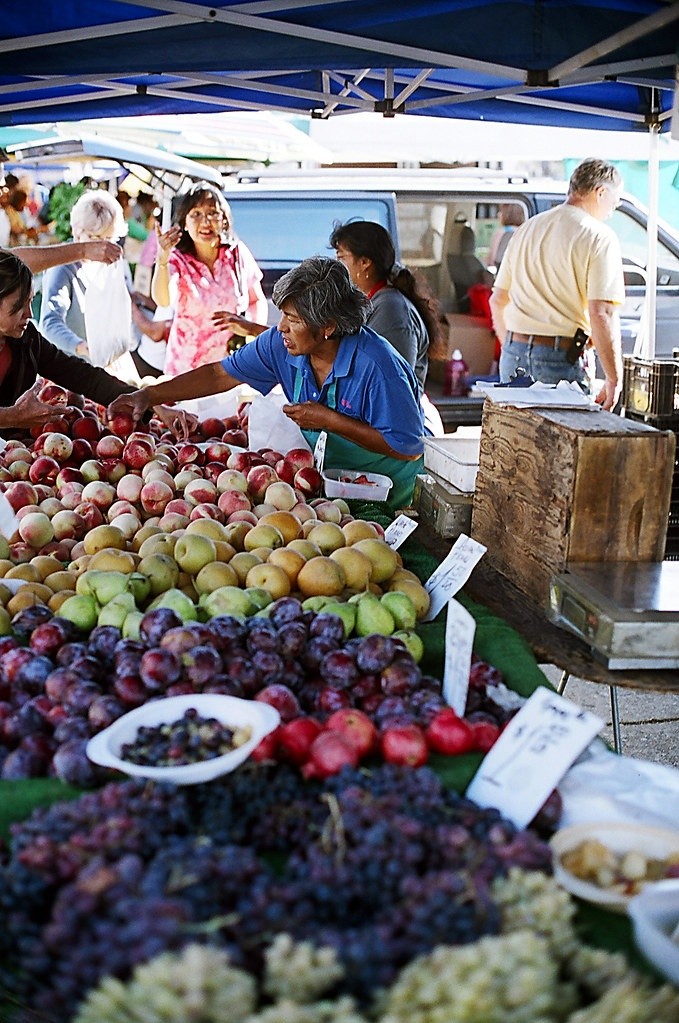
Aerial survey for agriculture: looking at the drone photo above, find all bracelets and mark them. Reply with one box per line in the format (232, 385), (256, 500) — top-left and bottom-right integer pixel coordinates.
(155, 259), (167, 268)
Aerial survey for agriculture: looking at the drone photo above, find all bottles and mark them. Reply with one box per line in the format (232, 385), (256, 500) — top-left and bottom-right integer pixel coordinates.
(443, 349), (468, 397)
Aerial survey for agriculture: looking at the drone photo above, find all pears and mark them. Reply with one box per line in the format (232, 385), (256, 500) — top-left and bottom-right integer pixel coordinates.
(0, 511), (423, 666)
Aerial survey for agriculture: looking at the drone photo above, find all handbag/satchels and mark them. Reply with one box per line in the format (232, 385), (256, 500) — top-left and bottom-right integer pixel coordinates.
(76, 252), (140, 368)
(247, 393), (312, 458)
(225, 245), (246, 356)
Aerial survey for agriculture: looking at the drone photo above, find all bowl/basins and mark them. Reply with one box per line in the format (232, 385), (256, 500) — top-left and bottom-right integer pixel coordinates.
(626, 878), (678, 984)
(548, 822), (679, 914)
(322, 468), (394, 500)
(84, 693), (281, 786)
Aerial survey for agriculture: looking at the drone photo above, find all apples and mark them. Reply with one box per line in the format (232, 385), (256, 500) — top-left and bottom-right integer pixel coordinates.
(253, 684), (501, 772)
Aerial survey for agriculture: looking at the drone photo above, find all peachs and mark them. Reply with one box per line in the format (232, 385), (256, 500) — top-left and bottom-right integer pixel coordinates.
(0, 385), (386, 558)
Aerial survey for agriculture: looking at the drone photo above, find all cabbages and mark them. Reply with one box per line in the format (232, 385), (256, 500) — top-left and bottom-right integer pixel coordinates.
(69, 190), (131, 244)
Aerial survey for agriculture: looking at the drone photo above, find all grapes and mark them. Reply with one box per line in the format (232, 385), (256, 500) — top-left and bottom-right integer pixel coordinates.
(0, 759), (679, 1023)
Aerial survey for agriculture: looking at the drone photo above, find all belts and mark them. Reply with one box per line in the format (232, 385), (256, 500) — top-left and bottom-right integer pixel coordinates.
(506, 331), (574, 349)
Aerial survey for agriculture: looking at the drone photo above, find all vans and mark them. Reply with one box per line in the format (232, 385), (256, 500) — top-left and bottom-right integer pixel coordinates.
(5, 135), (679, 427)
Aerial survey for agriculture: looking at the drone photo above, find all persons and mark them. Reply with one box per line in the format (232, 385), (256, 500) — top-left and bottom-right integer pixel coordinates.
(151, 183), (269, 376)
(0, 175), (36, 248)
(115, 191), (155, 240)
(329, 221), (448, 391)
(0, 247), (198, 441)
(489, 204), (524, 265)
(492, 159), (625, 410)
(41, 189), (166, 367)
(8, 241), (123, 274)
(106, 258), (433, 511)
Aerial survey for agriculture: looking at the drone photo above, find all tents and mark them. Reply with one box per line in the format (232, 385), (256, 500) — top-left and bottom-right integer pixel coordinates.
(1, 0), (679, 367)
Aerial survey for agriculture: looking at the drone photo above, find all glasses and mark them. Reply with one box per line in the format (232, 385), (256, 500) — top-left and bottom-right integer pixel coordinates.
(593, 182), (623, 211)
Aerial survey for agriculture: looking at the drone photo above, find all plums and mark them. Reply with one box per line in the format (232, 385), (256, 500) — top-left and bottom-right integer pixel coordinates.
(464, 658), (525, 724)
(0, 604), (445, 786)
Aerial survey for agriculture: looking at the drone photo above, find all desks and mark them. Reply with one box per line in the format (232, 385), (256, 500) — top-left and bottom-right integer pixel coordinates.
(404, 504), (679, 755)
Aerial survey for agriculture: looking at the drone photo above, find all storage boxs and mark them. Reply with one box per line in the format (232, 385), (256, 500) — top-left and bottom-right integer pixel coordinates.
(412, 354), (679, 608)
